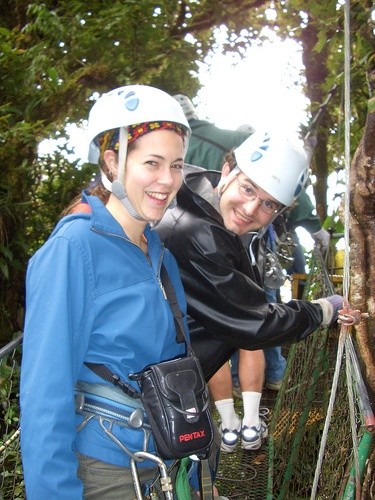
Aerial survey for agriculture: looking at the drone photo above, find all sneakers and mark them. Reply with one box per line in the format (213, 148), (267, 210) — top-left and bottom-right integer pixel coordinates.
(218, 413), (242, 452)
(241, 416), (268, 450)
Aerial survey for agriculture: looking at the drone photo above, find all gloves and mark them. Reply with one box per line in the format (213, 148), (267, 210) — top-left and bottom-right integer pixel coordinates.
(313, 294), (349, 329)
(311, 226), (331, 256)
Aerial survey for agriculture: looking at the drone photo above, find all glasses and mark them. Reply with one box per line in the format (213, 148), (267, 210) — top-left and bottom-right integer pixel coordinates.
(235, 177), (282, 217)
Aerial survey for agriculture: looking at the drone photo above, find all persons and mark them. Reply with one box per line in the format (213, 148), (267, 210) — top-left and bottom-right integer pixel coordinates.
(19, 85), (219, 500)
(150, 128), (348, 386)
(171, 93), (330, 453)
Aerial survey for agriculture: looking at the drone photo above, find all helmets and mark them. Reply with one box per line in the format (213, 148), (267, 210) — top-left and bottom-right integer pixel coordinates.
(235, 124), (309, 209)
(85, 85), (192, 166)
(171, 93), (199, 122)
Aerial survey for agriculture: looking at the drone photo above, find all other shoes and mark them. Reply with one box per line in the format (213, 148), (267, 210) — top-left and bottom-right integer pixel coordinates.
(266, 374), (292, 390)
(232, 386), (243, 399)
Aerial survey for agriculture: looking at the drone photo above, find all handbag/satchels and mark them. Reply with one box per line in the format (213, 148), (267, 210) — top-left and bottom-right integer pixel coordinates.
(129, 352), (214, 461)
